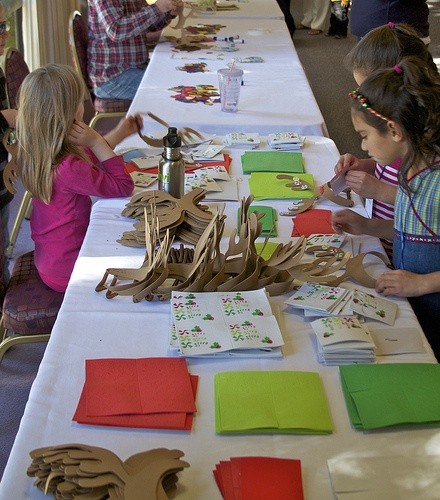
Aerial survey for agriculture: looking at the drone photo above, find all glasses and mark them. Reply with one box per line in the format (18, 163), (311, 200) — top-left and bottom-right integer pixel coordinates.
(0, 23), (11, 34)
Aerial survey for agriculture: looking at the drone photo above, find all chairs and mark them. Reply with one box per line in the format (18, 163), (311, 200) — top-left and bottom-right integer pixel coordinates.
(2, 47), (32, 256)
(68, 11), (127, 129)
(0, 249), (65, 363)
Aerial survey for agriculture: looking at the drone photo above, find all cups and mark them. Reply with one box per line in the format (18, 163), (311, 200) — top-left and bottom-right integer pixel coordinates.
(217, 68), (244, 113)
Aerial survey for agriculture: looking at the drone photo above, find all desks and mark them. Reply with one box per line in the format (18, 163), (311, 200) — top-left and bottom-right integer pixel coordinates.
(0, 137), (440, 500)
(127, 19), (331, 137)
(176, 0), (285, 19)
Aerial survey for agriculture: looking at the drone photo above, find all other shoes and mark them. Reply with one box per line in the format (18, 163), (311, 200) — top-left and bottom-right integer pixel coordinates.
(325, 33), (335, 36)
(295, 24), (311, 30)
(306, 30), (322, 35)
(334, 34), (347, 39)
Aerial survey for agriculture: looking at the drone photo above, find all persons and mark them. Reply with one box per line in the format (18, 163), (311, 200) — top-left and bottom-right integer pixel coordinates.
(335, 21), (435, 265)
(85, 0), (185, 101)
(0, 3), (17, 283)
(327, 57), (440, 296)
(17, 64), (143, 291)
(281, 0), (430, 51)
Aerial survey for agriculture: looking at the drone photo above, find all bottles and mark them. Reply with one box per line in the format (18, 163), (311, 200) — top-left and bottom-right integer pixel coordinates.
(158, 127), (185, 199)
